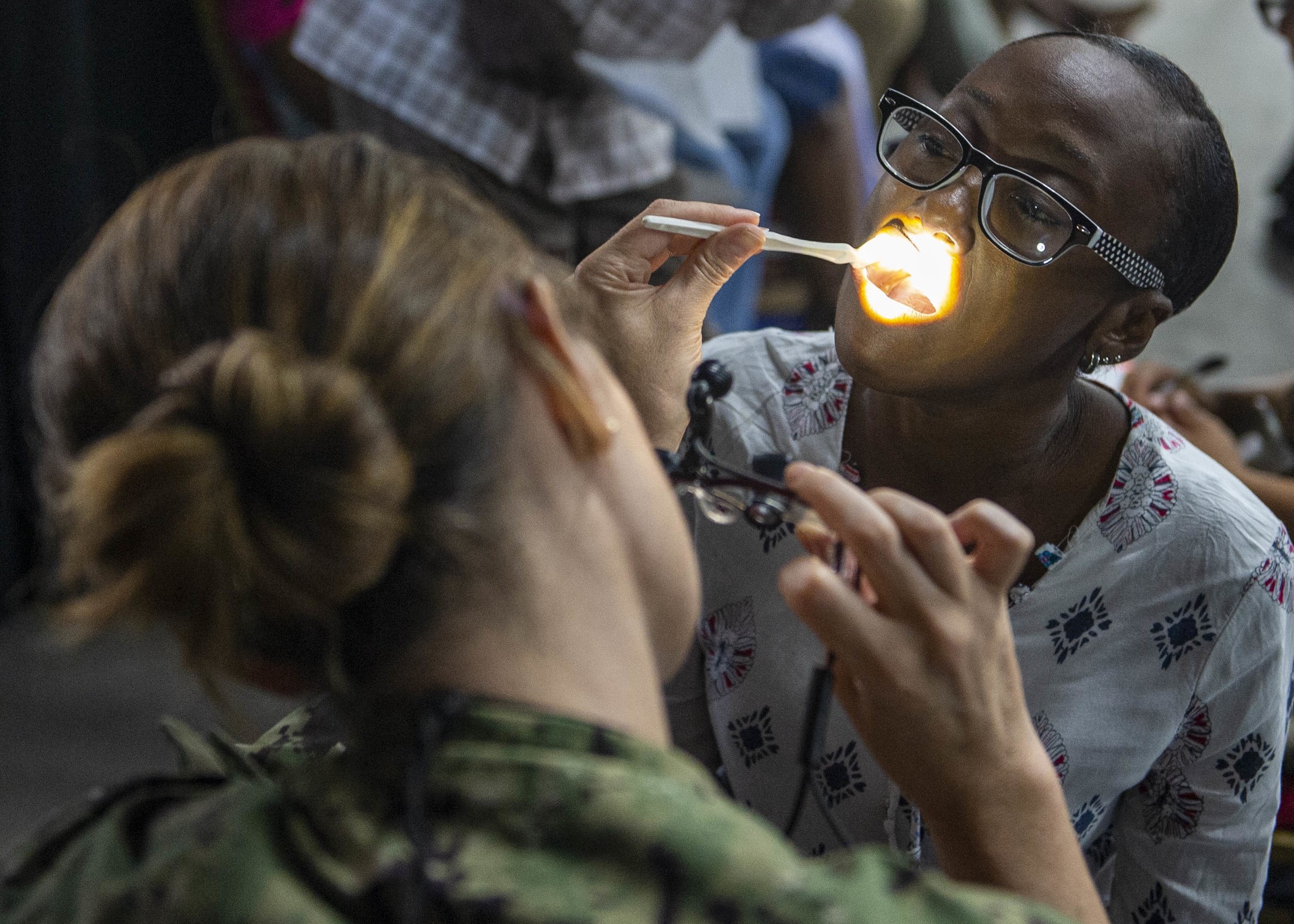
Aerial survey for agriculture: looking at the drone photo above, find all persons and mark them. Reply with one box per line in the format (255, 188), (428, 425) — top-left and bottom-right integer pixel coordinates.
(1, 137), (1113, 924)
(656, 31), (1294, 924)
(290, 3), (1294, 518)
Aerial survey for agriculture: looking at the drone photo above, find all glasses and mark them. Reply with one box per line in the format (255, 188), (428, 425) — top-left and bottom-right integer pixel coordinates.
(875, 86), (1168, 295)
